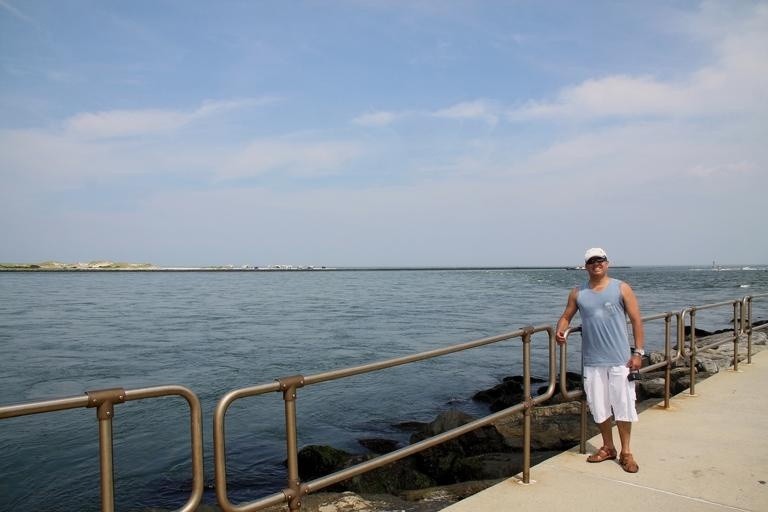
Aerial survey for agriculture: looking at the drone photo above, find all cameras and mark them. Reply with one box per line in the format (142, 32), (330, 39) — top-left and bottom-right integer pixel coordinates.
(627, 373), (642, 382)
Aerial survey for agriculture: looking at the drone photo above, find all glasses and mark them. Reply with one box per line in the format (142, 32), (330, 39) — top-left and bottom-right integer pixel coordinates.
(587, 258), (606, 264)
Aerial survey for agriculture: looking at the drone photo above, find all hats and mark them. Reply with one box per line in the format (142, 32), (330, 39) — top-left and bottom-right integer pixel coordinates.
(584, 247), (608, 264)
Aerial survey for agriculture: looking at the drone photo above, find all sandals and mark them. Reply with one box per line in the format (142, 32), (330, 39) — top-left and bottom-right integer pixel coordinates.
(618, 450), (639, 473)
(586, 446), (617, 463)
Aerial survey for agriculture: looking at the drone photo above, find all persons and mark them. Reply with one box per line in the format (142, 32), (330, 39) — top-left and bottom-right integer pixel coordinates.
(554, 246), (644, 473)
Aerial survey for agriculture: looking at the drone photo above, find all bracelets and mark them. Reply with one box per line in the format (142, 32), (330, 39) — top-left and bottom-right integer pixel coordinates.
(632, 349), (643, 358)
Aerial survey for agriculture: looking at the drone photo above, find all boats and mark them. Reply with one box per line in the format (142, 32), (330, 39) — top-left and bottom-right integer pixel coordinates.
(566, 265), (588, 270)
